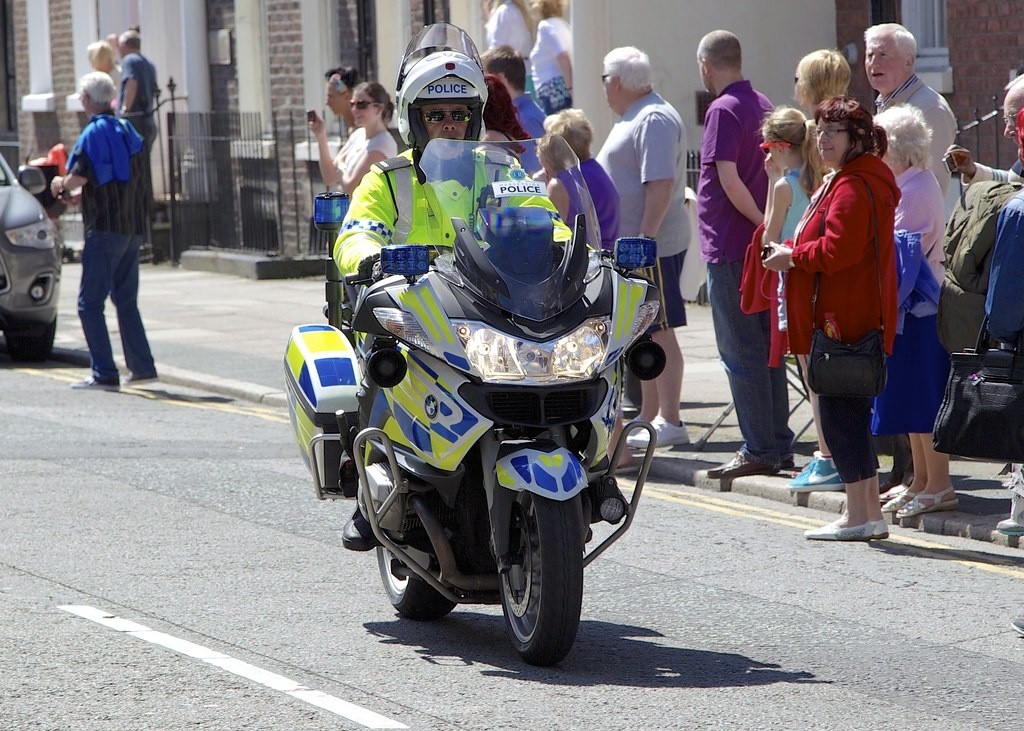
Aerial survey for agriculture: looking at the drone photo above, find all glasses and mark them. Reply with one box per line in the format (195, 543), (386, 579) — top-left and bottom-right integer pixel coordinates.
(601, 74), (609, 83)
(815, 126), (861, 137)
(759, 141), (790, 155)
(349, 100), (380, 109)
(1001, 115), (1018, 127)
(422, 107), (472, 124)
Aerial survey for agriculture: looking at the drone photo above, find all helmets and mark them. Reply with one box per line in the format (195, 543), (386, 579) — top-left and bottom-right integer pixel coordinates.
(396, 22), (488, 149)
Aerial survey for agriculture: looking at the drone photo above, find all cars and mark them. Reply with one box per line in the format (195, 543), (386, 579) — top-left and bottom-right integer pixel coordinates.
(1, 154), (61, 366)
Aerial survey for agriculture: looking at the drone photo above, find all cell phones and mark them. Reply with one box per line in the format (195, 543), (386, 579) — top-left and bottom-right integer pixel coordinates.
(761, 245), (775, 260)
(942, 152), (964, 174)
(308, 111), (316, 122)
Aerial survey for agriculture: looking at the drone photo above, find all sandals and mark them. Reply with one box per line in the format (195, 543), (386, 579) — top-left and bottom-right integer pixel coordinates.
(882, 489), (921, 512)
(895, 485), (958, 518)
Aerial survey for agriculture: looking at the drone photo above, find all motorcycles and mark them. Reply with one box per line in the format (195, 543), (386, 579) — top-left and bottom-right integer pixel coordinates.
(284, 132), (667, 664)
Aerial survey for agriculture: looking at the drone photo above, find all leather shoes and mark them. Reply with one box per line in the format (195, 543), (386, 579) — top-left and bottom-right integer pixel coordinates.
(706, 449), (795, 480)
(342, 508), (376, 551)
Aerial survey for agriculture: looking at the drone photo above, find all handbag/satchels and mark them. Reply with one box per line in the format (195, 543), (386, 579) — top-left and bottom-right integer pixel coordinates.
(805, 327), (885, 399)
(932, 315), (1024, 462)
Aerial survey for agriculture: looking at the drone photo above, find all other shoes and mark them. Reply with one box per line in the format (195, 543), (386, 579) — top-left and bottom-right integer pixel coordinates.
(804, 516), (873, 540)
(71, 376), (121, 392)
(869, 519), (889, 538)
(119, 368), (160, 385)
(880, 480), (912, 502)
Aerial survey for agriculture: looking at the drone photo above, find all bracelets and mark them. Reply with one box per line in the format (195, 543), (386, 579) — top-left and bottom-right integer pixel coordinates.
(61, 177), (70, 193)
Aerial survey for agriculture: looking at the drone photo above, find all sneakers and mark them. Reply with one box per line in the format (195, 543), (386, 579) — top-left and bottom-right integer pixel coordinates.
(786, 450), (845, 491)
(997, 520), (1024, 535)
(626, 415), (687, 447)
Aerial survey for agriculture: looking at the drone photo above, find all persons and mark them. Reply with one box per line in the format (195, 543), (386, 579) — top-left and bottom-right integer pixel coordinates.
(943, 69), (1024, 537)
(86, 30), (158, 235)
(694, 30), (796, 479)
(595, 47), (690, 448)
(761, 24), (958, 540)
(332, 23), (629, 552)
(51, 72), (159, 389)
(306, 0), (622, 471)
